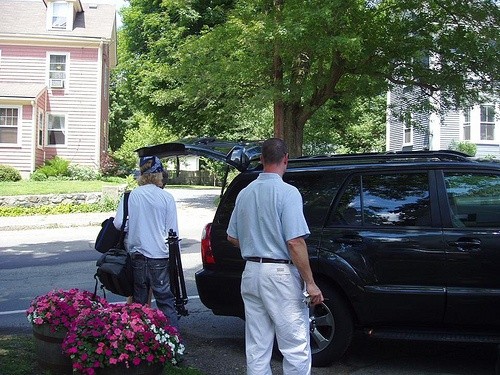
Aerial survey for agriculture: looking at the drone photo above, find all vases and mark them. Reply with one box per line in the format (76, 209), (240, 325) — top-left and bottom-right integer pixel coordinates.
(96, 358), (164, 375)
(32, 322), (73, 371)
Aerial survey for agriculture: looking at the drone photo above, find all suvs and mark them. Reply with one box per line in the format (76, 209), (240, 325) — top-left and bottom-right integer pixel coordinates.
(134, 136), (500, 367)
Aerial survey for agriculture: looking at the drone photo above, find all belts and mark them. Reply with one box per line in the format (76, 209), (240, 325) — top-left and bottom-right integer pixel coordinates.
(247, 256), (294, 264)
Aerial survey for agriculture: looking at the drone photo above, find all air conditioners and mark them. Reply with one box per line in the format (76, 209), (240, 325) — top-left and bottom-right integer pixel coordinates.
(50, 79), (63, 88)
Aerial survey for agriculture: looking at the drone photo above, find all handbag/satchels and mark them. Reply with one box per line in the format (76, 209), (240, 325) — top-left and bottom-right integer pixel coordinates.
(94, 217), (126, 254)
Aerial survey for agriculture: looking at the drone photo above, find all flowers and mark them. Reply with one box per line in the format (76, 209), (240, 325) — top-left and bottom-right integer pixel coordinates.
(26, 287), (108, 328)
(61, 301), (185, 375)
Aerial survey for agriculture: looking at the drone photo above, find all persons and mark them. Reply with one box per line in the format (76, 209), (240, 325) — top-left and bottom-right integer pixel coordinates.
(114, 155), (181, 330)
(226, 139), (324, 375)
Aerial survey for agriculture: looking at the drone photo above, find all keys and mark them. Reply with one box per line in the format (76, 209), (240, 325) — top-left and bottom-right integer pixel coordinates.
(302, 292), (312, 307)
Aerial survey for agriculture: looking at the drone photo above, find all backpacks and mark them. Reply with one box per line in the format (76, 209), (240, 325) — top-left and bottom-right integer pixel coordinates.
(97, 248), (133, 297)
